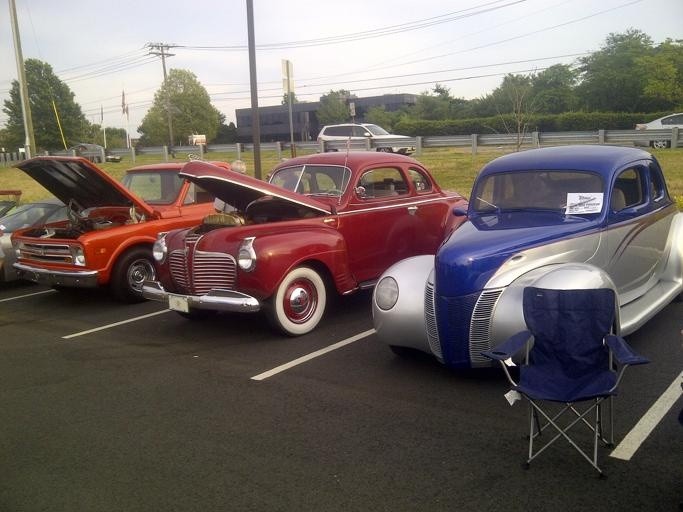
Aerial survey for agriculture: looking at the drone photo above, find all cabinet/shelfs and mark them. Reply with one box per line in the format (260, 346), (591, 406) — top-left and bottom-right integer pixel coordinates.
(366, 183), (398, 196)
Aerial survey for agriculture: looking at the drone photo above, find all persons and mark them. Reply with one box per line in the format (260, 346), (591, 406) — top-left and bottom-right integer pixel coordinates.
(212, 159), (247, 214)
(0, 224), (6, 270)
(277, 157), (304, 195)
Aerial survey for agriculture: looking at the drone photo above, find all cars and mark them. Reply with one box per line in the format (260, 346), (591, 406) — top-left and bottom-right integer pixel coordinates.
(632, 112), (683, 148)
(373, 142), (683, 366)
(8, 156), (232, 302)
(0, 198), (65, 285)
(141, 151), (468, 337)
(0, 189), (22, 220)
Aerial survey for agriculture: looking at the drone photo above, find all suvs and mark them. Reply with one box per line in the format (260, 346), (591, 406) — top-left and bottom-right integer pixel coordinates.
(316, 122), (415, 155)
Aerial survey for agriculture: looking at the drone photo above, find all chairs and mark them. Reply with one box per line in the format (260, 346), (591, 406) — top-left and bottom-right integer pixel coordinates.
(611, 189), (626, 212)
(480, 287), (651, 479)
(516, 177), (547, 205)
